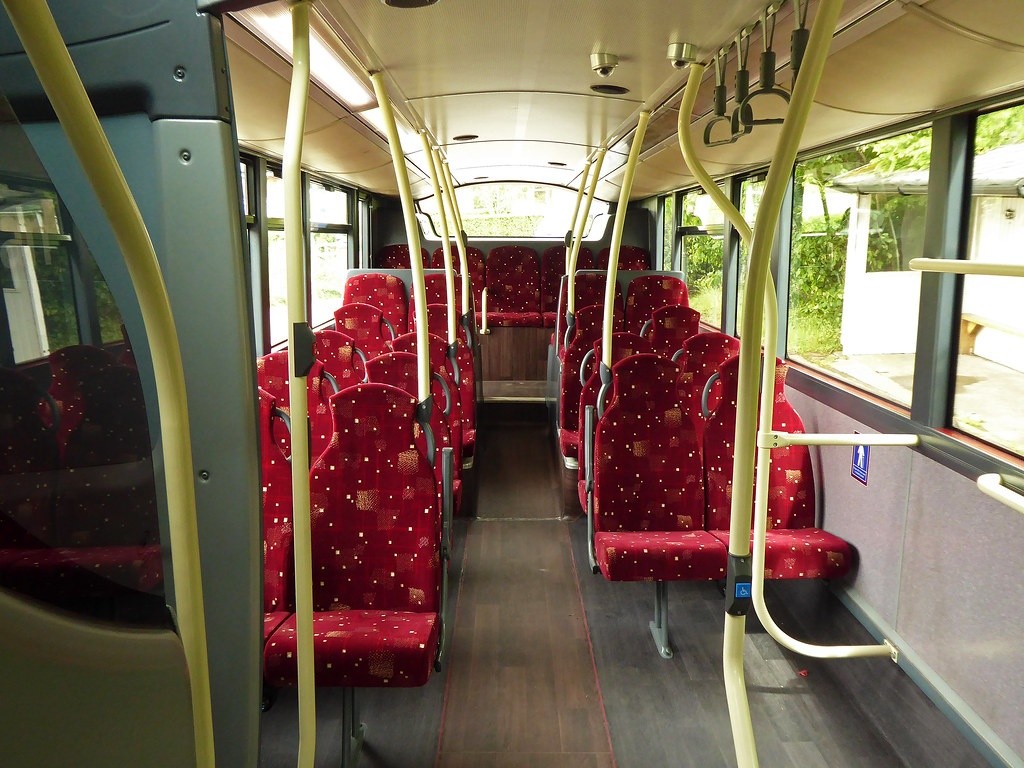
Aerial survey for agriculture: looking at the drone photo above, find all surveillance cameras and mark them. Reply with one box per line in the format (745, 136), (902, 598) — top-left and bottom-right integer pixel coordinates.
(667, 43), (690, 69)
(590, 53), (618, 78)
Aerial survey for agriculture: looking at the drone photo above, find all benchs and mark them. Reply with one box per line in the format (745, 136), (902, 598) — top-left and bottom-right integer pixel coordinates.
(258, 244), (856, 768)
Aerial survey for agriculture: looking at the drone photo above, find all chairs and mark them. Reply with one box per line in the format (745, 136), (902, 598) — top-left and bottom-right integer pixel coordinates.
(0, 322), (166, 628)
